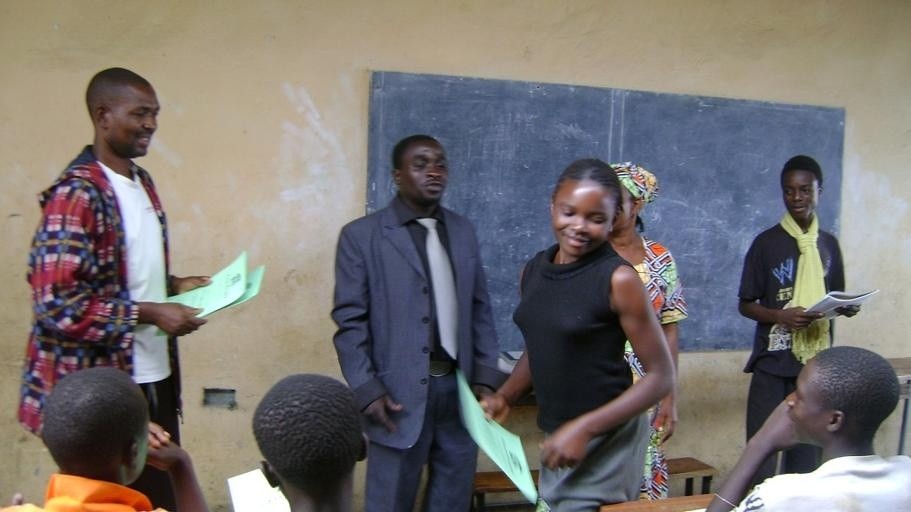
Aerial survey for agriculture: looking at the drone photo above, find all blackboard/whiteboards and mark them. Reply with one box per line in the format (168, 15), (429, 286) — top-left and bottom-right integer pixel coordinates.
(365, 71), (846, 353)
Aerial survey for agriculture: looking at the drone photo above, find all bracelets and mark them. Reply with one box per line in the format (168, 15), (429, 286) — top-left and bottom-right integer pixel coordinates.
(713, 491), (737, 508)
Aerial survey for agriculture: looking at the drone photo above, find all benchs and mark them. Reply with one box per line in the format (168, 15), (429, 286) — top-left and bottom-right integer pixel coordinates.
(469, 456), (715, 510)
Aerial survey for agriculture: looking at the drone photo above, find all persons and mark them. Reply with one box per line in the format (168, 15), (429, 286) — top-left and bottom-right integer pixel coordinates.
(481, 159), (676, 512)
(605, 161), (687, 499)
(738, 156), (882, 500)
(18, 67), (211, 507)
(704, 346), (911, 512)
(329, 134), (499, 512)
(0, 370), (368, 512)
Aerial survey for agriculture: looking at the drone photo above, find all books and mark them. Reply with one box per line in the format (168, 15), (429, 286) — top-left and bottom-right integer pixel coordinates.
(805, 288), (881, 328)
(153, 251), (264, 336)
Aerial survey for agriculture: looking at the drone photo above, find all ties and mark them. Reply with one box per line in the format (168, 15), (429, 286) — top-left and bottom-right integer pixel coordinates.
(414, 217), (462, 360)
